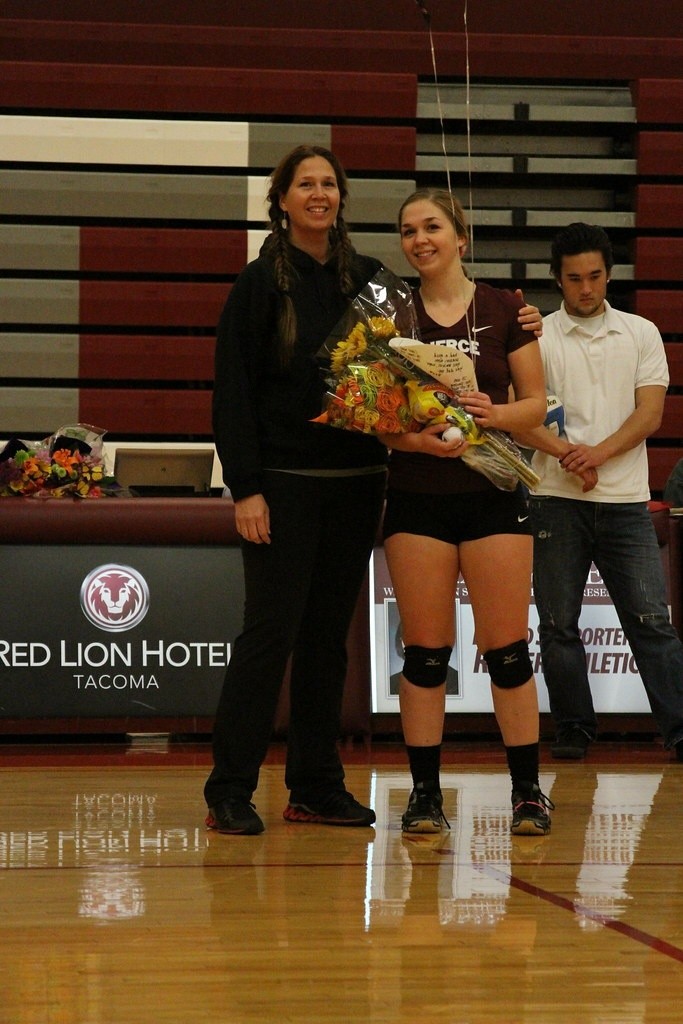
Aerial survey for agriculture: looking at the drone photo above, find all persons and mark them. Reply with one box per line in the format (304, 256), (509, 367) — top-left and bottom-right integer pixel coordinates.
(509, 221), (683, 765)
(203, 145), (542, 835)
(376, 190), (548, 834)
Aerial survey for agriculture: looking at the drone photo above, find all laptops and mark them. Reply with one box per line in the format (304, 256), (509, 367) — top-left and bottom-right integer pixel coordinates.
(114, 448), (215, 498)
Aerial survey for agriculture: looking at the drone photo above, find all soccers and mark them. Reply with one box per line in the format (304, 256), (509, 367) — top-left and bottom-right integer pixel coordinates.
(511, 388), (565, 451)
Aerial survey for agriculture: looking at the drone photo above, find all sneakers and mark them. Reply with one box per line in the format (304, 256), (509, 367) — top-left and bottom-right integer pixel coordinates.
(283, 788), (376, 825)
(400, 779), (451, 832)
(550, 726), (589, 758)
(511, 781), (557, 835)
(205, 796), (265, 833)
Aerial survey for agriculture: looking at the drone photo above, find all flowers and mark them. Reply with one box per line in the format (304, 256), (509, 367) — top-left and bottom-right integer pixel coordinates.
(304, 309), (550, 500)
(0, 429), (113, 500)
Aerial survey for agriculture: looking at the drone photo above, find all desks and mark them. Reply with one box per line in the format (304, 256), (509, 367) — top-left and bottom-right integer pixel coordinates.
(0, 498), (372, 748)
